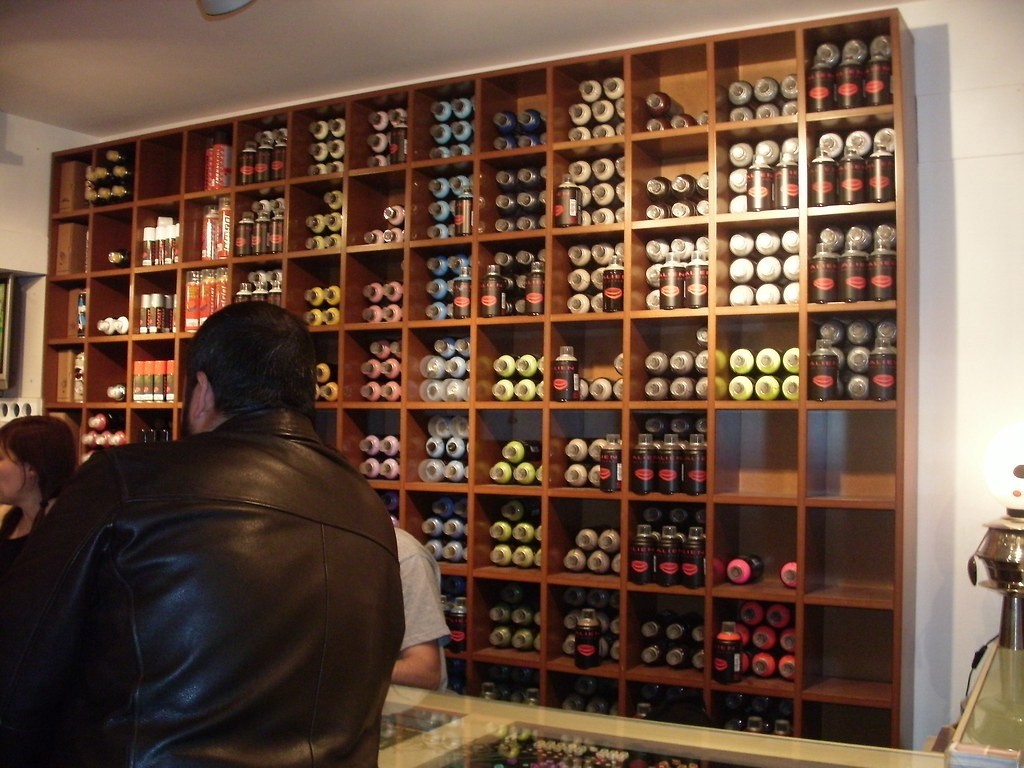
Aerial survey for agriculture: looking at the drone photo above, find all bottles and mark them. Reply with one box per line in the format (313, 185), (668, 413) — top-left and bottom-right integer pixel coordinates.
(713, 692), (793, 738)
(551, 673), (619, 718)
(712, 598), (796, 686)
(69, 36), (899, 501)
(639, 619), (706, 673)
(633, 505), (708, 590)
(562, 527), (623, 574)
(558, 587), (622, 669)
(780, 562), (798, 587)
(434, 576), (467, 653)
(488, 499), (542, 569)
(373, 488), (399, 526)
(418, 495), (468, 565)
(727, 553), (764, 584)
(483, 582), (541, 652)
(473, 664), (542, 708)
(633, 685), (705, 726)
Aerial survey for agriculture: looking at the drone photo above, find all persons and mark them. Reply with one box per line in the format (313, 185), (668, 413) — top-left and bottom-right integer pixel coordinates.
(386, 519), (451, 695)
(0, 299), (408, 768)
(0, 414), (80, 671)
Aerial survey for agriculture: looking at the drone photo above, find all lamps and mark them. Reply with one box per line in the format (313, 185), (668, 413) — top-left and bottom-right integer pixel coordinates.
(967, 462), (1024, 650)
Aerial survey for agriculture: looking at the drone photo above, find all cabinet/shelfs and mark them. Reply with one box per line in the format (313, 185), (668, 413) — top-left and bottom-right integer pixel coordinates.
(39, 6), (918, 749)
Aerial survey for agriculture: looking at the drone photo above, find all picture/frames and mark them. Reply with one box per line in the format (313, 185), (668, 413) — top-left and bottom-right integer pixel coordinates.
(0, 272), (15, 390)
